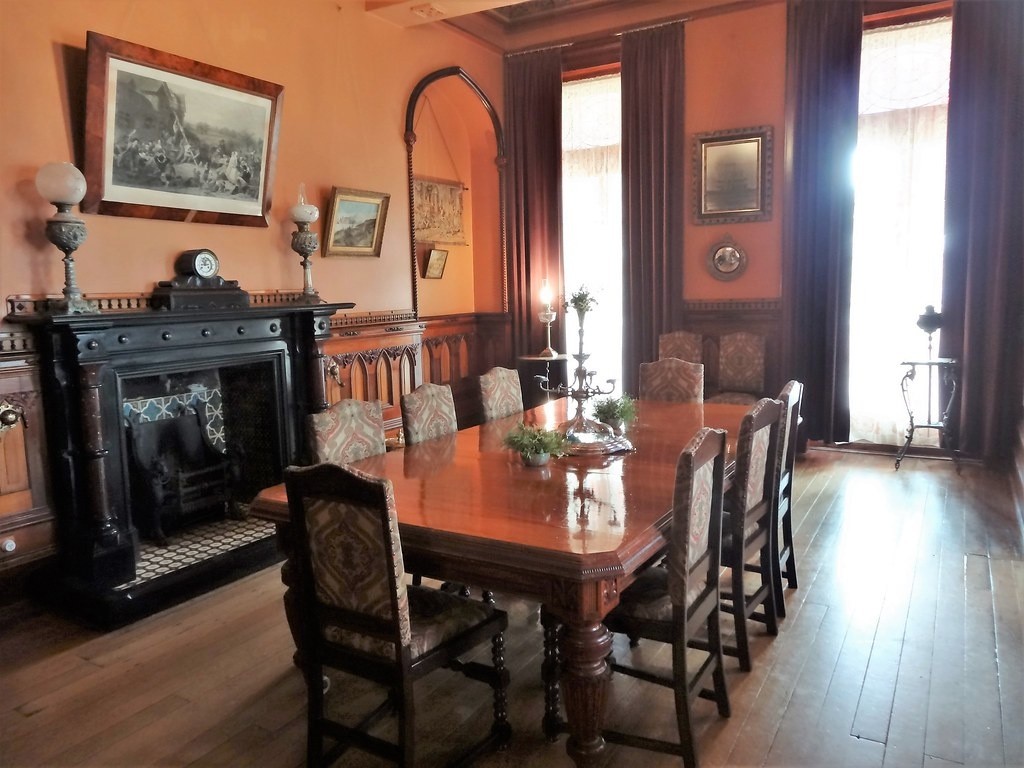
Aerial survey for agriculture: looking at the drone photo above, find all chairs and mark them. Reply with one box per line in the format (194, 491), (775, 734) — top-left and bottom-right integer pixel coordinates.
(604, 426), (730, 767)
(719, 398), (784, 671)
(639, 358), (703, 403)
(658, 329), (703, 364)
(773, 380), (806, 620)
(704, 331), (765, 405)
(281, 462), (507, 768)
(476, 367), (523, 421)
(400, 383), (457, 446)
(310, 397), (386, 466)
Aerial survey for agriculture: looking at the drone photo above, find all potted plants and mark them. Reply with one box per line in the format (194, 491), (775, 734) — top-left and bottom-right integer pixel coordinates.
(501, 422), (568, 466)
(561, 283), (598, 352)
(595, 392), (641, 426)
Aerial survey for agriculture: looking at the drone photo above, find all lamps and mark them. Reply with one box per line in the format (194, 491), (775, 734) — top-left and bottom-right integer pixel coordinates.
(535, 288), (559, 358)
(289, 180), (328, 305)
(35, 158), (101, 314)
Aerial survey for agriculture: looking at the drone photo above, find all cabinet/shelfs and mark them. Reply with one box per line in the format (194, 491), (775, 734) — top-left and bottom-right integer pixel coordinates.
(889, 359), (966, 475)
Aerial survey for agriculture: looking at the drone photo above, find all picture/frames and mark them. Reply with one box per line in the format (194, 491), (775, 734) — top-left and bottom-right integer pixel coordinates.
(690, 123), (773, 226)
(321, 185), (391, 258)
(422, 249), (449, 279)
(80, 31), (283, 228)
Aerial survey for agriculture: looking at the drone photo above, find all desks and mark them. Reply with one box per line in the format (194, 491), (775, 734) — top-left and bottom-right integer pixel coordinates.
(249, 396), (756, 768)
(518, 354), (571, 401)
